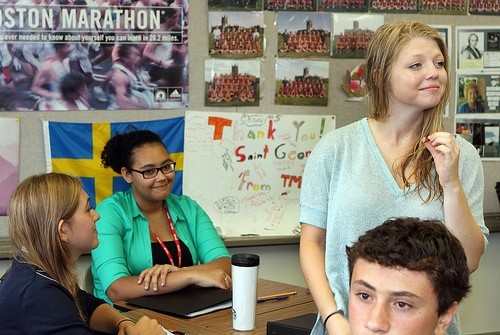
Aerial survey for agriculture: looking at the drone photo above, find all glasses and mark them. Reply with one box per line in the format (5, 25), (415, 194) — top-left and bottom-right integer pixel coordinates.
(126, 160), (176, 179)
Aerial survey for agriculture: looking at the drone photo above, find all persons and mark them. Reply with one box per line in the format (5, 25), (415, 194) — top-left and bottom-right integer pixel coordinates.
(0, 172), (167, 335)
(0, 0), (486, 114)
(298, 21), (492, 335)
(90, 130), (232, 307)
(345, 216), (472, 335)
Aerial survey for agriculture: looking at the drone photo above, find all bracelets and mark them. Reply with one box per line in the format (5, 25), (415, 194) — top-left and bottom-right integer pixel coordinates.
(323, 310), (344, 332)
(117, 319), (136, 331)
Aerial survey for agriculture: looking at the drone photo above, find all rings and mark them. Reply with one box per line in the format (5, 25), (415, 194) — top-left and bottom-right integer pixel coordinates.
(224, 278), (228, 280)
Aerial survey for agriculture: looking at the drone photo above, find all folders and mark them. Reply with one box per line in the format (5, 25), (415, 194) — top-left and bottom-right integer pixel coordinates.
(125, 283), (234, 320)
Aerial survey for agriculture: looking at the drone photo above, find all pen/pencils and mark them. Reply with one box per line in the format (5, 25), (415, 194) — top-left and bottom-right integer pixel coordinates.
(242, 234), (259, 237)
(256, 290), (297, 303)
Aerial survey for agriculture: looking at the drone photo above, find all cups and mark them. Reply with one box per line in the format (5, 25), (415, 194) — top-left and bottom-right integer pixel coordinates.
(231, 253), (260, 331)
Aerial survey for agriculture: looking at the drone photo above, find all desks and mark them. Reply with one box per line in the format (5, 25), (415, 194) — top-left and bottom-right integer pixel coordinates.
(112, 278), (318, 335)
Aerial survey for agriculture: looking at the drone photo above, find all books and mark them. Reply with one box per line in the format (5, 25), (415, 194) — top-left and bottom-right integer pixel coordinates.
(126, 284), (232, 318)
